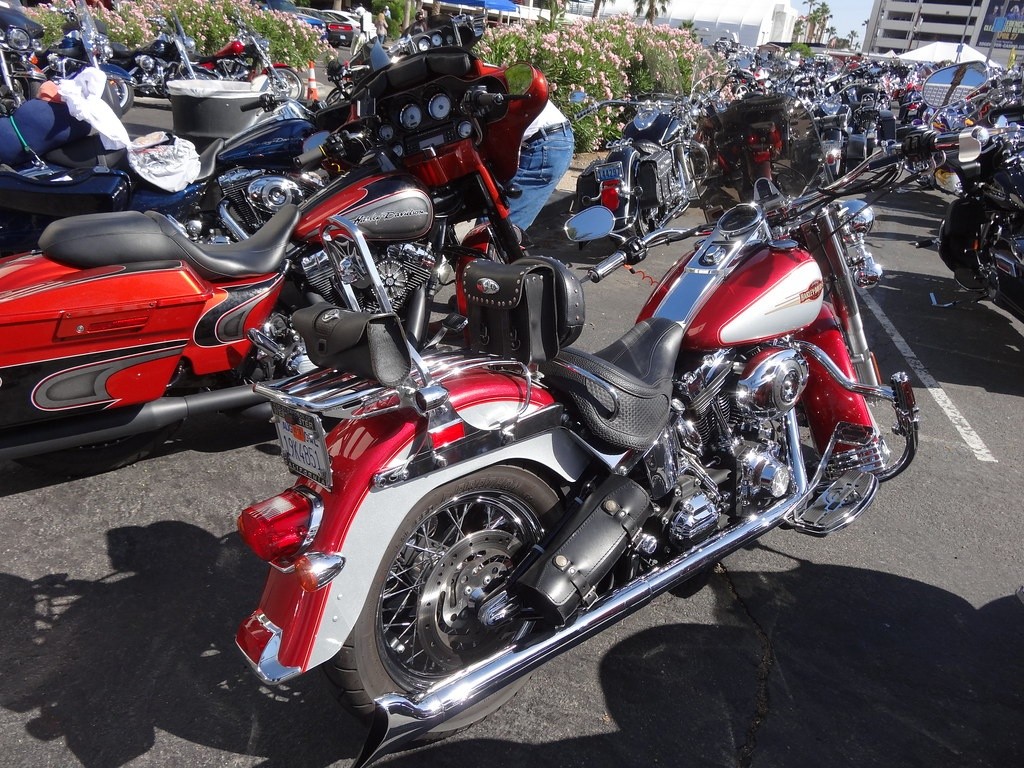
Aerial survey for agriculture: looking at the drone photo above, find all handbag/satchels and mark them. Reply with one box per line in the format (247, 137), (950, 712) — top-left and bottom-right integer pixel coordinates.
(291, 301), (410, 385)
(127, 130), (201, 193)
(462, 258), (560, 364)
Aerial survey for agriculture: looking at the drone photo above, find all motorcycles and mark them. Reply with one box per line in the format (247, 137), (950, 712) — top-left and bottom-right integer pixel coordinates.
(230, 149), (922, 768)
(0, 1), (1024, 470)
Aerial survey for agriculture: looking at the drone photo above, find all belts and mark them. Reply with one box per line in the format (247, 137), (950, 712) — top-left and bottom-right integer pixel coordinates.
(525, 123), (570, 144)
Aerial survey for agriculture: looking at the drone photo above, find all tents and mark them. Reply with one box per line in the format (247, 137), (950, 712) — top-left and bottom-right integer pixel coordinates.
(863, 50), (899, 59)
(438, 0), (516, 25)
(900, 42), (999, 68)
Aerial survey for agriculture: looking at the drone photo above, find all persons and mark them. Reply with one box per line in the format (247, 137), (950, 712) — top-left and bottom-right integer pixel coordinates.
(475, 99), (575, 232)
(986, 5), (1024, 27)
(377, 12), (388, 44)
(383, 6), (390, 18)
(355, 3), (366, 16)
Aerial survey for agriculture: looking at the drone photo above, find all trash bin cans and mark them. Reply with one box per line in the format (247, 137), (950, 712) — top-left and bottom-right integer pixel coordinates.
(166, 79), (274, 156)
